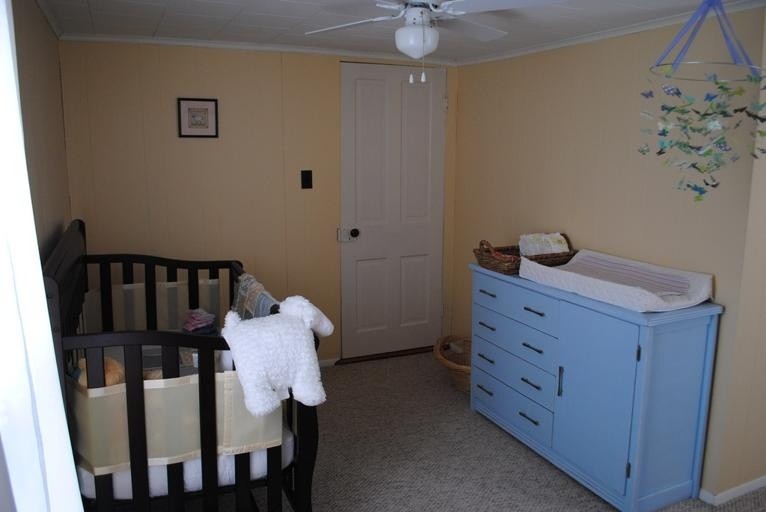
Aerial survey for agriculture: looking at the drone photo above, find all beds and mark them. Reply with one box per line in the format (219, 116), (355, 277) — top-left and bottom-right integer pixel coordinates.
(40, 213), (324, 511)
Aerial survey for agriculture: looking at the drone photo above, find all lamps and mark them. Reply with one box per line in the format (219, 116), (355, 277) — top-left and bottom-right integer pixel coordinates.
(395, 3), (446, 87)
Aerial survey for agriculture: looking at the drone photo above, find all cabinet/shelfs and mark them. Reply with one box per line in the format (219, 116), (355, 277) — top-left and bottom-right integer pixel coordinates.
(467, 262), (564, 465)
(549, 290), (724, 512)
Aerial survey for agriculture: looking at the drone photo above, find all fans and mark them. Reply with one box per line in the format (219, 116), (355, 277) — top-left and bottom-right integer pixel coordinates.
(298, 0), (509, 48)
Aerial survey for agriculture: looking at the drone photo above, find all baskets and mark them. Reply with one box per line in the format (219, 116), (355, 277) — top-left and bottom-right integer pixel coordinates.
(429, 330), (474, 396)
(469, 227), (582, 278)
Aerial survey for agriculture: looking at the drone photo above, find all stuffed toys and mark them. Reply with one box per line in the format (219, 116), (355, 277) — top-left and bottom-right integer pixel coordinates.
(78, 356), (165, 391)
(224, 294), (334, 416)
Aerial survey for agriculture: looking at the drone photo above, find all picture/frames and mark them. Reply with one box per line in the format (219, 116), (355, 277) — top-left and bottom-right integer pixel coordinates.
(175, 97), (220, 139)
